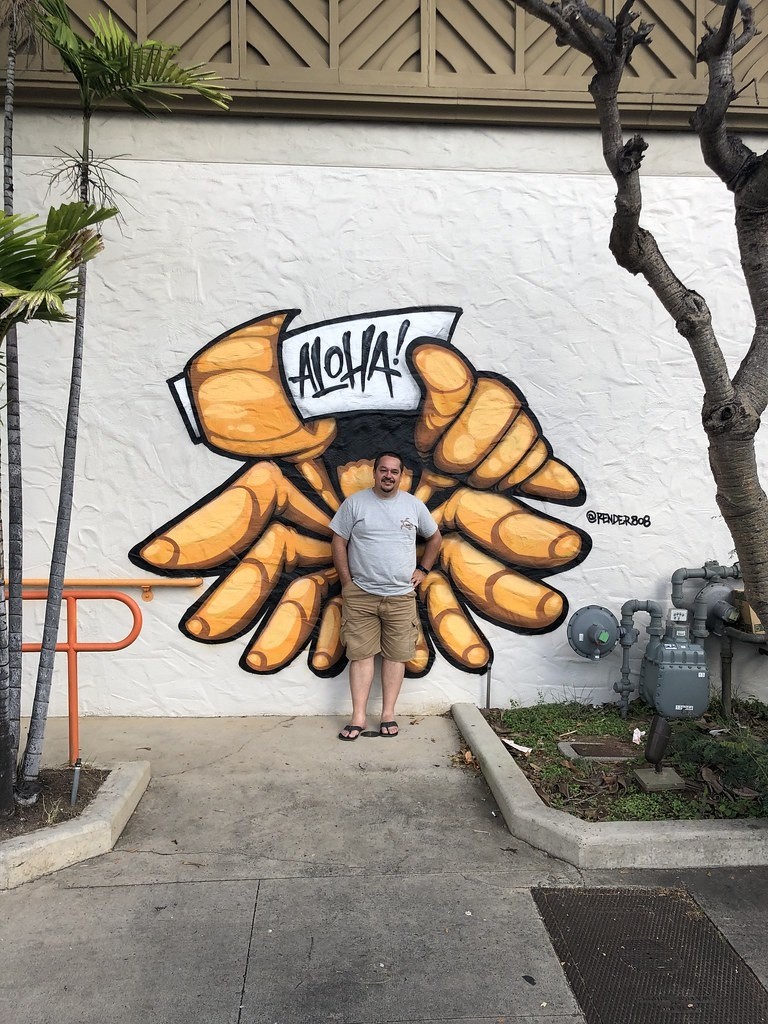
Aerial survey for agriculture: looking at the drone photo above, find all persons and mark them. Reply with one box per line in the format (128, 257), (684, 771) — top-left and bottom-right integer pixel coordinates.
(327, 451), (443, 741)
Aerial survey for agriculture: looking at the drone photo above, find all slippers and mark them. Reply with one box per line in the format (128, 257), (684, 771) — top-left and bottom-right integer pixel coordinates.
(379, 721), (399, 737)
(338, 725), (365, 741)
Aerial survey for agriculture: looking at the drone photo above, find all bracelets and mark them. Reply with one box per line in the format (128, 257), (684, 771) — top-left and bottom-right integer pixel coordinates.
(417, 564), (429, 575)
